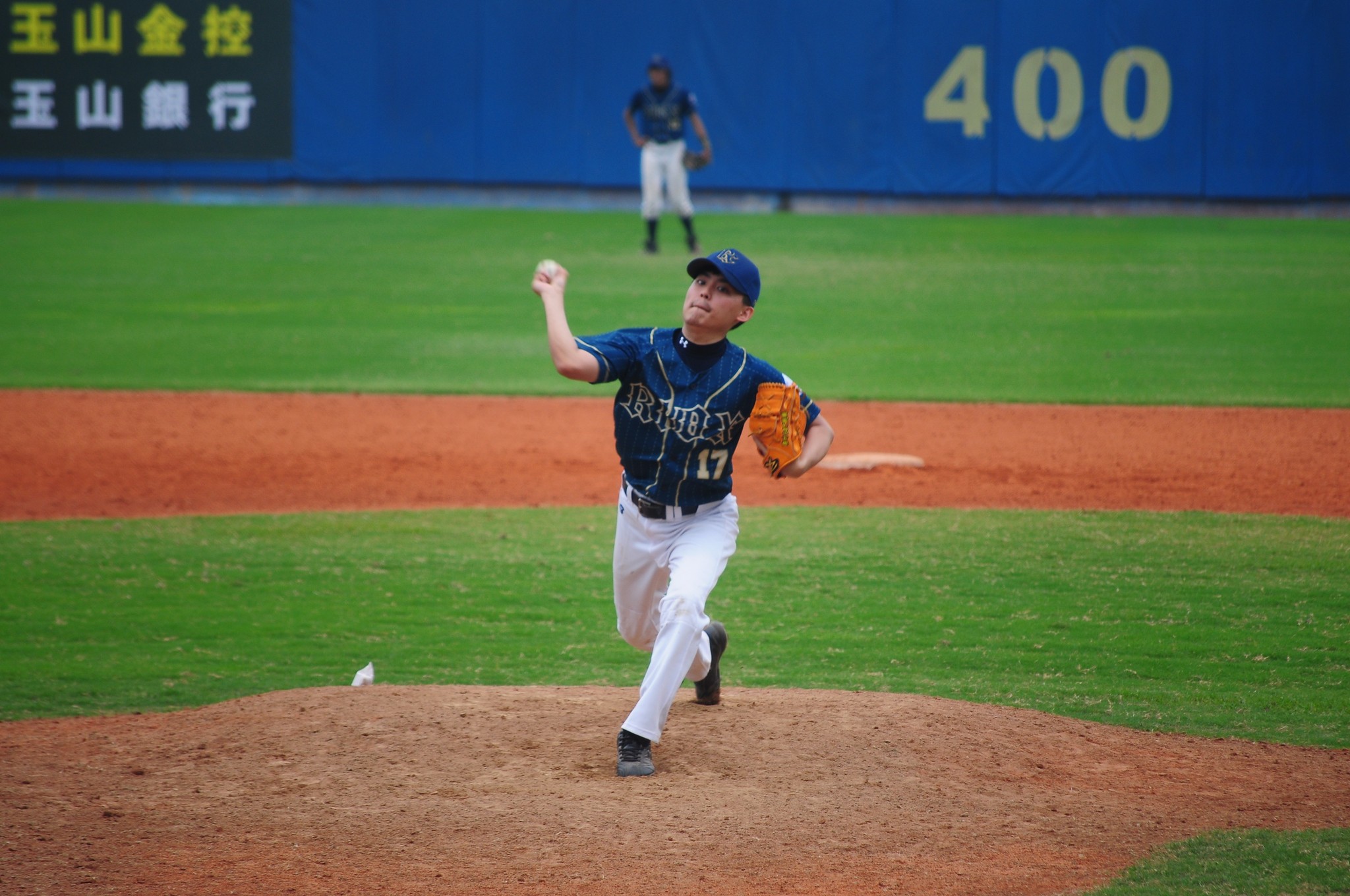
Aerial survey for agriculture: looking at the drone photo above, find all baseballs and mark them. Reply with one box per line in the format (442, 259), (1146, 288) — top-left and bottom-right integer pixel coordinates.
(535, 258), (560, 278)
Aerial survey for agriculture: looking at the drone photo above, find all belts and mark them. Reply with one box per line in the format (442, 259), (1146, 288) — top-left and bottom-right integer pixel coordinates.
(622, 473), (698, 519)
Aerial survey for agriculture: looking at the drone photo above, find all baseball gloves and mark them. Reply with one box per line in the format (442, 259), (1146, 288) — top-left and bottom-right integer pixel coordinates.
(748, 382), (807, 480)
(680, 151), (708, 170)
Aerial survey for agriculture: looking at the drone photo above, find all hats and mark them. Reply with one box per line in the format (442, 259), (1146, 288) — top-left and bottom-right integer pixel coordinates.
(686, 248), (761, 311)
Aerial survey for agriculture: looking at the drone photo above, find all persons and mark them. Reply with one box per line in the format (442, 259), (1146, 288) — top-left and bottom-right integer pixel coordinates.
(529, 249), (834, 778)
(623, 55), (712, 256)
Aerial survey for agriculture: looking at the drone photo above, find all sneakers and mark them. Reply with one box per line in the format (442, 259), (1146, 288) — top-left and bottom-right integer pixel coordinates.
(693, 622), (727, 705)
(615, 729), (656, 776)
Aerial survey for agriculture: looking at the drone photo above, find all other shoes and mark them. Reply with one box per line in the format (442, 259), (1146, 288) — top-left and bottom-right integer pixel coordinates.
(686, 236), (697, 252)
(644, 236), (656, 253)
